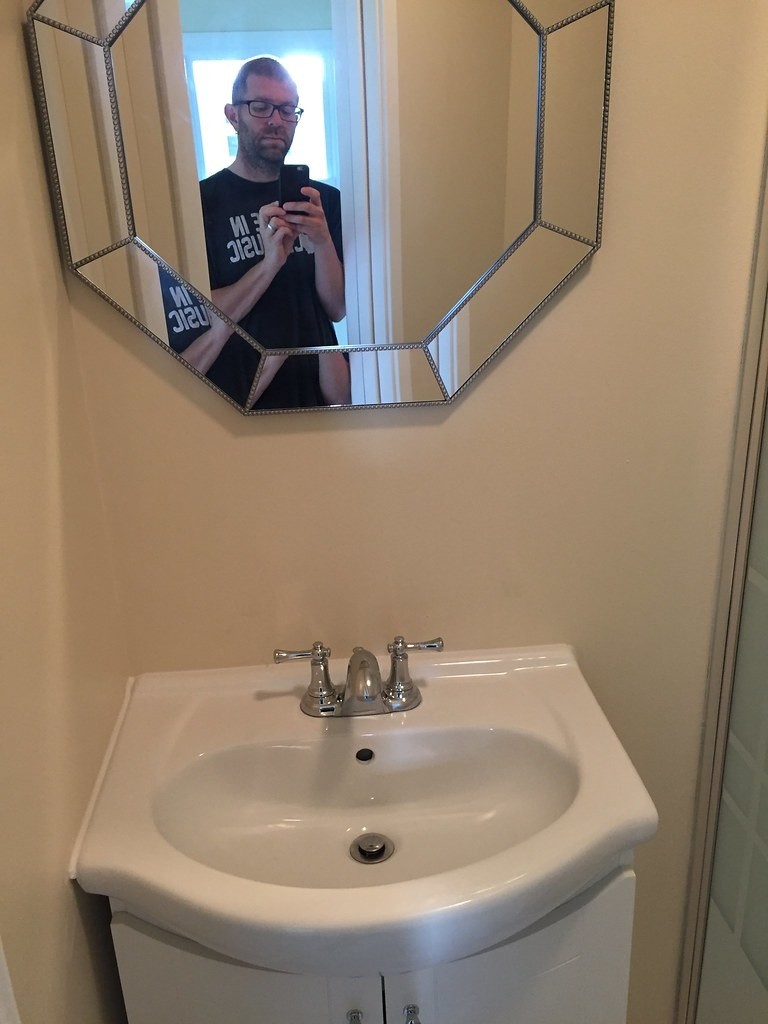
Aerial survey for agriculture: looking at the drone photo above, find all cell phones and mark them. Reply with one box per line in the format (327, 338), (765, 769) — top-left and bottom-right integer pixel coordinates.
(279, 165), (310, 218)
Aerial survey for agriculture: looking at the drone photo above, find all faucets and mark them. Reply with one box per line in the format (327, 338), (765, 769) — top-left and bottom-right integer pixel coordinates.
(344, 645), (385, 718)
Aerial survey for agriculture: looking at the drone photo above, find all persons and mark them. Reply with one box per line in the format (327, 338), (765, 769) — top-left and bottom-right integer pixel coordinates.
(178, 312), (354, 409)
(181, 56), (346, 347)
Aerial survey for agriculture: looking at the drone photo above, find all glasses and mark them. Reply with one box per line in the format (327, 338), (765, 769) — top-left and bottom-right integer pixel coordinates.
(233, 100), (304, 123)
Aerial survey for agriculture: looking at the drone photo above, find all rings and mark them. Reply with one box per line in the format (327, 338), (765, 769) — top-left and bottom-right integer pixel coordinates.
(266, 222), (276, 234)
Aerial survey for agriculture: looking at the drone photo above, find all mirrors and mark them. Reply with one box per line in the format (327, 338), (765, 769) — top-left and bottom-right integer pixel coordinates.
(24, 0), (615, 417)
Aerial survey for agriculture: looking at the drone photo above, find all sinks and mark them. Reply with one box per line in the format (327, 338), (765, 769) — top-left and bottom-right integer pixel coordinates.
(148, 724), (581, 889)
